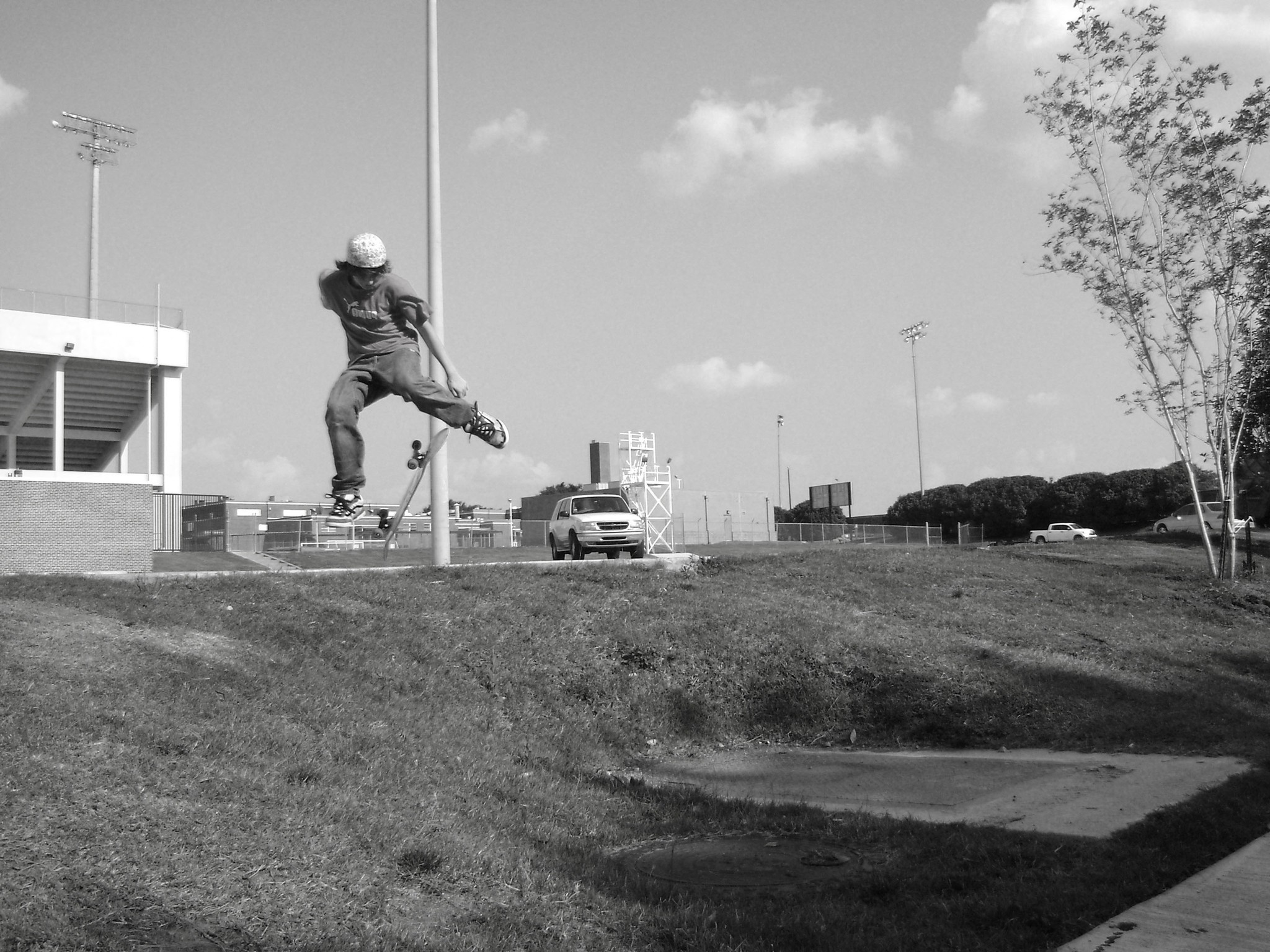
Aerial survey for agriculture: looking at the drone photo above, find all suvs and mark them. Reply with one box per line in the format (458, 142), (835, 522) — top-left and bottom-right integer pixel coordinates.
(549, 494), (645, 560)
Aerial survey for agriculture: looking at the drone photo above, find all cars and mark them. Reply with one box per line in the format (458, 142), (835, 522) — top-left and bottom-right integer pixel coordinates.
(1153, 500), (1230, 534)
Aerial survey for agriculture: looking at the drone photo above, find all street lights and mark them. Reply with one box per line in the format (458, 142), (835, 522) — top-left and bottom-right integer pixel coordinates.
(766, 497), (771, 541)
(53, 111), (138, 320)
(704, 495), (710, 544)
(777, 414), (784, 508)
(897, 316), (930, 498)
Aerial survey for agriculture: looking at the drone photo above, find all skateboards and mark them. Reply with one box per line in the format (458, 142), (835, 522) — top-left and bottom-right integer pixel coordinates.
(375, 428), (451, 560)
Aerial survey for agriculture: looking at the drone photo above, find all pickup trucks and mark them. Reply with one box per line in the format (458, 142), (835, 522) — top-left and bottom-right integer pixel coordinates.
(1029, 522), (1098, 544)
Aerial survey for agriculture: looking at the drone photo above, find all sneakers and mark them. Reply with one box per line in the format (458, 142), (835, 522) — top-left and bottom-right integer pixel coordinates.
(325, 494), (366, 527)
(462, 401), (509, 449)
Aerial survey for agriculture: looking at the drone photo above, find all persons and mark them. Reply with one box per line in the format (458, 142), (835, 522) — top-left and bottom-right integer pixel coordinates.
(319, 231), (510, 528)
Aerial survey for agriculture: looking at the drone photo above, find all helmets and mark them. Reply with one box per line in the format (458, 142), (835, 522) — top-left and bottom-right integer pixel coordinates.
(347, 233), (387, 268)
